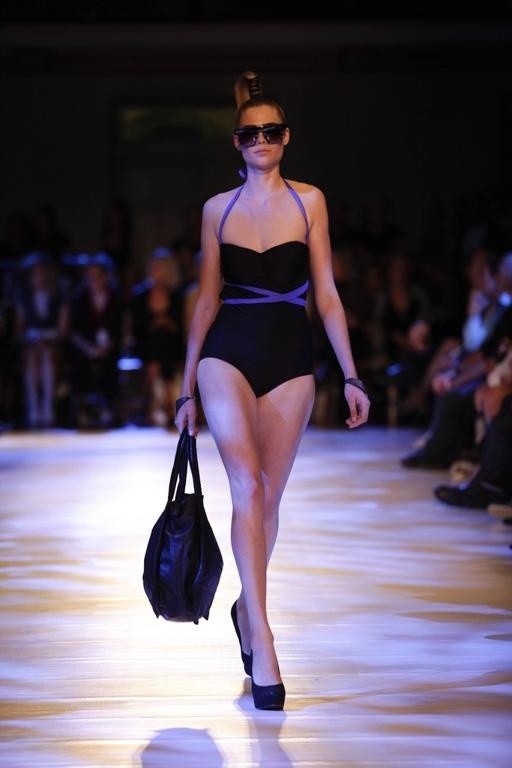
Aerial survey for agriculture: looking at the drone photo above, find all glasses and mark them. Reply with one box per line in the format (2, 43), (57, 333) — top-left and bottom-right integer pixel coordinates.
(234, 124), (287, 147)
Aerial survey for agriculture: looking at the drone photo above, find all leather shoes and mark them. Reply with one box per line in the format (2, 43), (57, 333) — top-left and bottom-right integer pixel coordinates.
(403, 447), (452, 469)
(435, 482), (489, 506)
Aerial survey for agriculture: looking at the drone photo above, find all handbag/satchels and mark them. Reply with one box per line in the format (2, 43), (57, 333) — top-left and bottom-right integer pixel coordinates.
(142, 427), (224, 625)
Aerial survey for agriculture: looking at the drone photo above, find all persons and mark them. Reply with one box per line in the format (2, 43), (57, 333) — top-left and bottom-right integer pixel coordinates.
(4, 242), (434, 430)
(172, 68), (375, 713)
(401, 243), (512, 547)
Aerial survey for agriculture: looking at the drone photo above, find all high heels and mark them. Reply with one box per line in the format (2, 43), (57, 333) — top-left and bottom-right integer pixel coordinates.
(231, 599), (286, 709)
